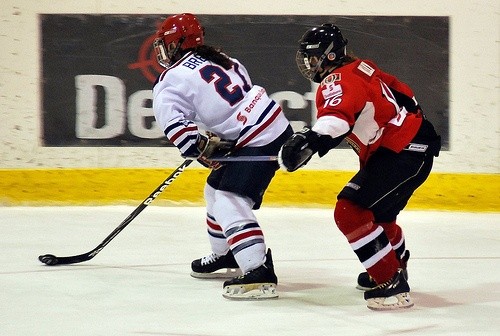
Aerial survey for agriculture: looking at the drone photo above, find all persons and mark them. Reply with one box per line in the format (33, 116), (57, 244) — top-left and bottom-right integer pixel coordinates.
(277, 24), (442, 311)
(152, 14), (294, 299)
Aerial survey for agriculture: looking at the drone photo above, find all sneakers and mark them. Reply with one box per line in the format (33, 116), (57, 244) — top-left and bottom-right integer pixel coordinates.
(362, 268), (413, 311)
(355, 250), (411, 291)
(219, 247), (278, 301)
(191, 249), (243, 280)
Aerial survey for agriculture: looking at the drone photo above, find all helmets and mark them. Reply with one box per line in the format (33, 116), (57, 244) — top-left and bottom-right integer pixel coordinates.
(152, 13), (205, 68)
(295, 21), (350, 84)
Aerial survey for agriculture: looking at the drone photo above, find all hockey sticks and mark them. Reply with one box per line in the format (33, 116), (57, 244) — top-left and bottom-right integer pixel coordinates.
(38, 158), (194, 267)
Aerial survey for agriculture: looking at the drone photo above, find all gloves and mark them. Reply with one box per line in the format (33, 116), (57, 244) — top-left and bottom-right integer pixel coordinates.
(278, 127), (321, 171)
(206, 141), (236, 165)
